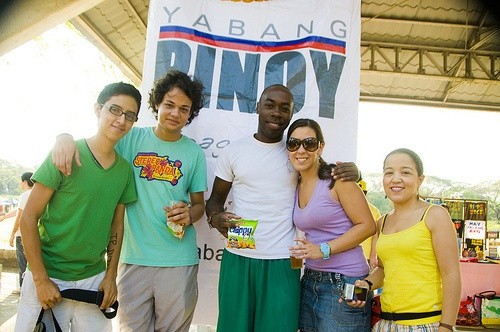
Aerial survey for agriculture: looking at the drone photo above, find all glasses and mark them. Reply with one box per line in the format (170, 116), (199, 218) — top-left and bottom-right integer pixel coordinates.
(285, 137), (321, 153)
(101, 102), (139, 123)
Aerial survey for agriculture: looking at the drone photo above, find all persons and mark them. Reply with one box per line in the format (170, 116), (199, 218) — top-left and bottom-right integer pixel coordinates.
(337, 148), (462, 332)
(12, 82), (135, 332)
(0, 171), (39, 294)
(205, 85), (371, 332)
(51, 68), (209, 332)
(286, 116), (375, 332)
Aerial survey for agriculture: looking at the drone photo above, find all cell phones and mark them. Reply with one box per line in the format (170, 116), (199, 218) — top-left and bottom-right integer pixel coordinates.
(344, 283), (368, 302)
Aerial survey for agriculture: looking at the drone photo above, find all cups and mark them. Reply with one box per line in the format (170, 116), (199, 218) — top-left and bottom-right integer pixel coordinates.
(289, 247), (303, 269)
(167, 200), (184, 223)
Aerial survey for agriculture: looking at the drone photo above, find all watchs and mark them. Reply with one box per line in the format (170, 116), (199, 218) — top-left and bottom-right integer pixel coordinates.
(319, 242), (331, 261)
(439, 322), (456, 332)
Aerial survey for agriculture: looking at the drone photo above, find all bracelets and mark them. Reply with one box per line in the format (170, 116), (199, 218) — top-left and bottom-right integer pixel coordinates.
(207, 210), (221, 229)
(357, 171), (363, 182)
(362, 278), (373, 290)
